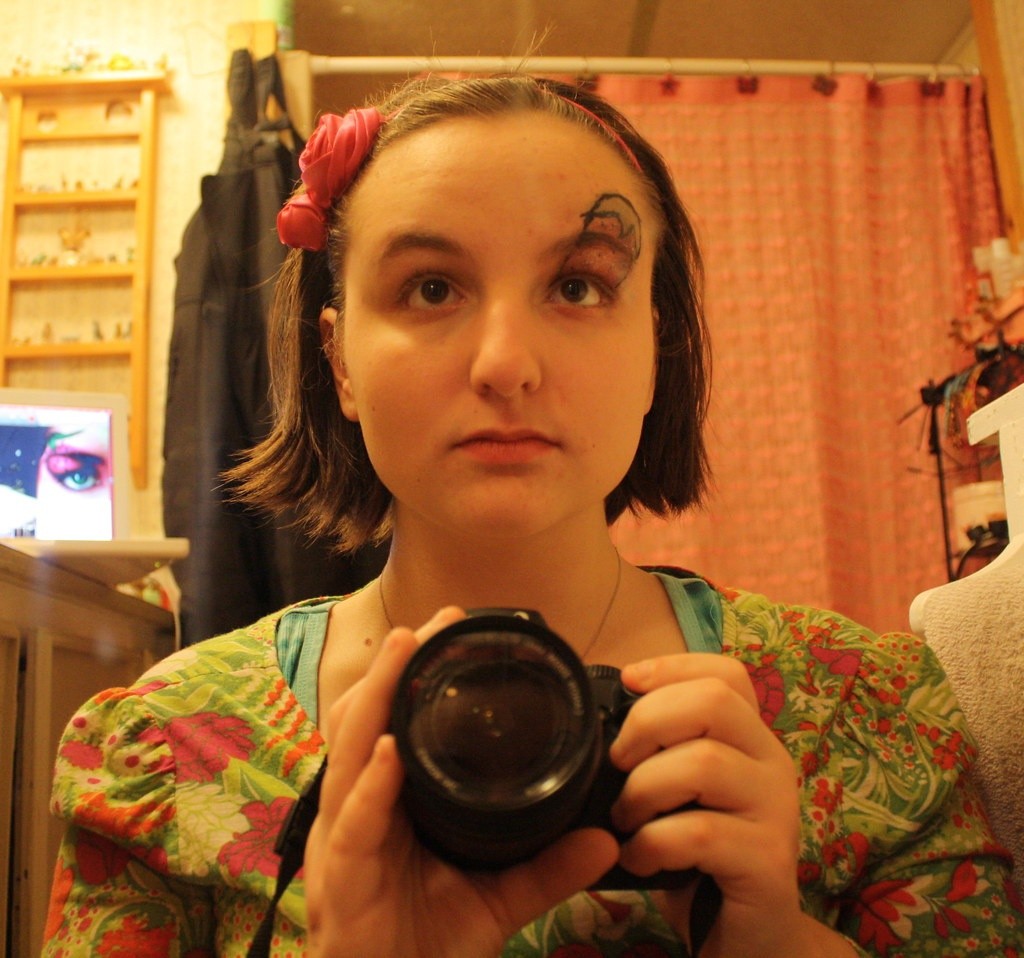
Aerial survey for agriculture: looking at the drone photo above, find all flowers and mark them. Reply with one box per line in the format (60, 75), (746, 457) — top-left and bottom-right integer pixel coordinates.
(276, 109), (381, 250)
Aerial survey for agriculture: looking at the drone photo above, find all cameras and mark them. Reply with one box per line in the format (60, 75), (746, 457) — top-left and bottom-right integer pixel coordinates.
(375, 606), (696, 892)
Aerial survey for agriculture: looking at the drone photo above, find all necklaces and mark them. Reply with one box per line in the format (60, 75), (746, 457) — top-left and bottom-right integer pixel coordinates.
(380, 545), (621, 658)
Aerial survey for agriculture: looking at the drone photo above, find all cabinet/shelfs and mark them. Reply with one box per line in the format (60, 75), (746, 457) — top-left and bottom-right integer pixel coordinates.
(928, 345), (1024, 582)
(0, 543), (176, 958)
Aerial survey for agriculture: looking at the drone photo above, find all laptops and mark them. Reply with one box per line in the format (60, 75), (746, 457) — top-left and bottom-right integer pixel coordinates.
(1, 387), (193, 590)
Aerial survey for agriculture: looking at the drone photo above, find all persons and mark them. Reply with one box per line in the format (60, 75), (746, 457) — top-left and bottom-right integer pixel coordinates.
(35, 414), (112, 539)
(37, 78), (1024, 958)
